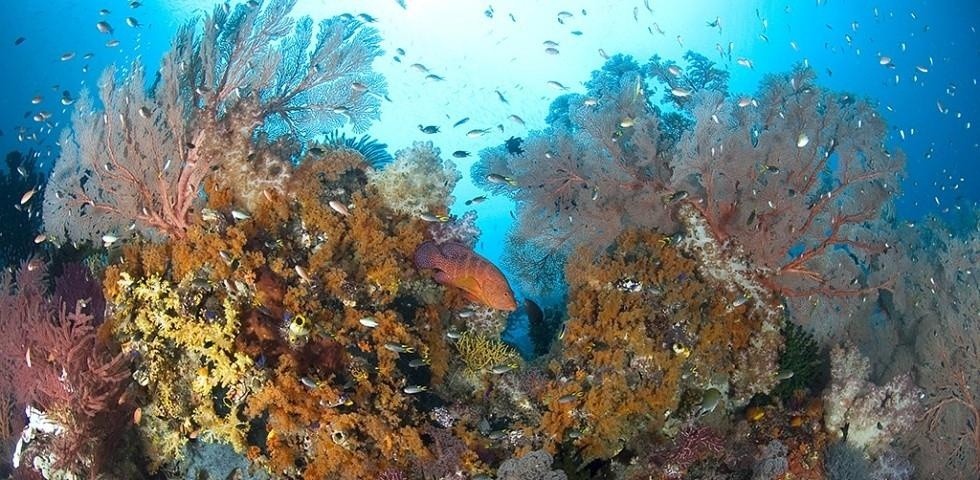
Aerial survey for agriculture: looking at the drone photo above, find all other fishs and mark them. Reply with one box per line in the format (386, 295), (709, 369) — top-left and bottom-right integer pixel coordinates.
(14, 1), (980, 443)
